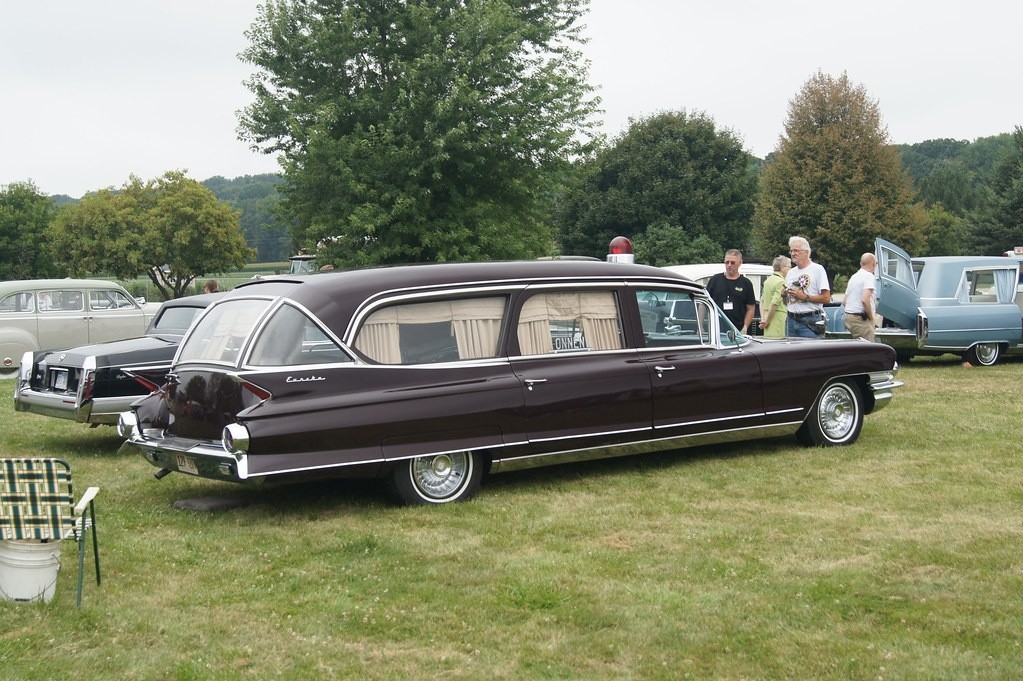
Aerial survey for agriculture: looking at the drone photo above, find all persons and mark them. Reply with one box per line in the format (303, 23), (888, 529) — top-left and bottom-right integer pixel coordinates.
(28, 293), (52, 310)
(203, 279), (218, 294)
(759, 255), (791, 337)
(780, 236), (831, 337)
(696, 249), (756, 336)
(842, 253), (876, 343)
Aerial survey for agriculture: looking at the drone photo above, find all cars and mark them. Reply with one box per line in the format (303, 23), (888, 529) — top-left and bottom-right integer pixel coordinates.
(574, 263), (774, 337)
(1, 279), (163, 368)
(115, 259), (903, 506)
(823, 238), (1023, 366)
(13, 291), (349, 430)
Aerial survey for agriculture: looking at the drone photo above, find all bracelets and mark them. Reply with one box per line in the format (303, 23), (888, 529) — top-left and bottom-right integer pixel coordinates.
(805, 295), (809, 302)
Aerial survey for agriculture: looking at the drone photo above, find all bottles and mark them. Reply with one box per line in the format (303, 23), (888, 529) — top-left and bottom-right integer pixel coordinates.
(789, 281), (801, 303)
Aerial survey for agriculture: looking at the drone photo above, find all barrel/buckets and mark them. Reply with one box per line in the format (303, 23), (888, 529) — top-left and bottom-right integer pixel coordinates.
(0, 540), (62, 603)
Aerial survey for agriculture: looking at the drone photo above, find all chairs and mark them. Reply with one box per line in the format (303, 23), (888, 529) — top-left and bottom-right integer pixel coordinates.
(0, 457), (100, 609)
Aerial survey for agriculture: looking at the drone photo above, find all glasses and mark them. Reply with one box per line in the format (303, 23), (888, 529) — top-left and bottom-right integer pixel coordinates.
(789, 250), (806, 253)
(724, 261), (737, 265)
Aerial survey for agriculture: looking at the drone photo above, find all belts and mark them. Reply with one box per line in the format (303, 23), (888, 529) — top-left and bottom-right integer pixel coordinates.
(845, 312), (862, 315)
(788, 310), (823, 318)
